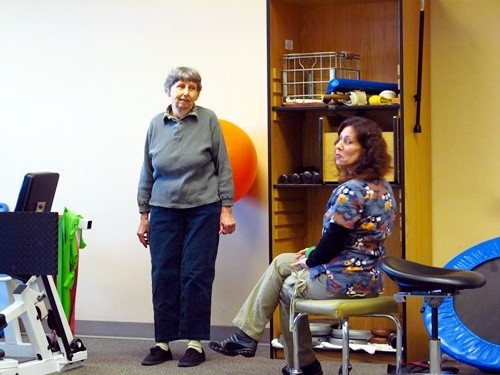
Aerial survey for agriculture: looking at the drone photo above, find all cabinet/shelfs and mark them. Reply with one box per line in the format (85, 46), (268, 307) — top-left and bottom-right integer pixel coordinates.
(265, 0), (433, 364)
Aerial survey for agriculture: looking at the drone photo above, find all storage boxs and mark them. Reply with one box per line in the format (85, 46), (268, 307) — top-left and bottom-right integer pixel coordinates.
(318, 113), (399, 184)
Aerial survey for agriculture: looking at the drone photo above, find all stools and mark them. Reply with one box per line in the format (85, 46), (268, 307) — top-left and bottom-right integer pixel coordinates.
(379, 255), (490, 375)
(291, 295), (404, 375)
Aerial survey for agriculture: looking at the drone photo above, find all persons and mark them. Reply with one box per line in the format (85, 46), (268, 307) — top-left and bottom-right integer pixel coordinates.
(209, 116), (397, 375)
(137, 67), (237, 367)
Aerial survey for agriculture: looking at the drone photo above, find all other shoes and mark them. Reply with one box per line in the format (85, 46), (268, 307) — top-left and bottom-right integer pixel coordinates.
(141, 346), (172, 365)
(208, 334), (256, 358)
(178, 348), (205, 367)
(282, 360), (324, 375)
